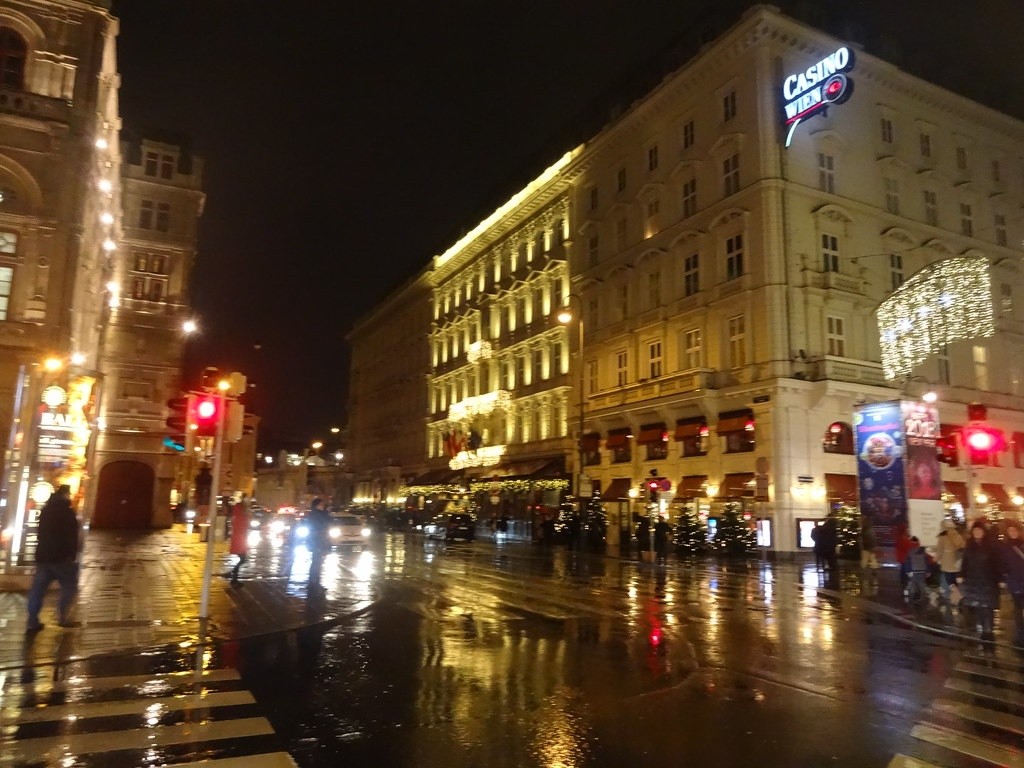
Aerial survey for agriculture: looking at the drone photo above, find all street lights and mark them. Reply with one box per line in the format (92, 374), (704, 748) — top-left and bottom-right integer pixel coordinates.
(558, 294), (585, 499)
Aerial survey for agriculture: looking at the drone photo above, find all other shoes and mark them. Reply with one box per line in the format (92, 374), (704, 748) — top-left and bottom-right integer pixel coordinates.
(28, 623), (44, 634)
(59, 622), (81, 628)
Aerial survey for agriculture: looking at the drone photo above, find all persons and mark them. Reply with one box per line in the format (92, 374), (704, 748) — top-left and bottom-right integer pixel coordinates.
(487, 513), (838, 572)
(225, 492), (253, 586)
(222, 496), (232, 537)
(306, 497), (333, 593)
(23, 484), (82, 632)
(892, 517), (1024, 640)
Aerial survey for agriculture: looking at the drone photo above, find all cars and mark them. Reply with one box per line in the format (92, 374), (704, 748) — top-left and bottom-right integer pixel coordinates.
(213, 495), (372, 552)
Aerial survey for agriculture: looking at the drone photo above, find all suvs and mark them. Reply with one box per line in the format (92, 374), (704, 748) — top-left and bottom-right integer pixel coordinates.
(423, 511), (474, 543)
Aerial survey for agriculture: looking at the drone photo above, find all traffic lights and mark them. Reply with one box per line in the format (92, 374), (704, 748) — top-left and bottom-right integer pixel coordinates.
(186, 390), (219, 440)
(963, 402), (992, 470)
(165, 398), (194, 457)
